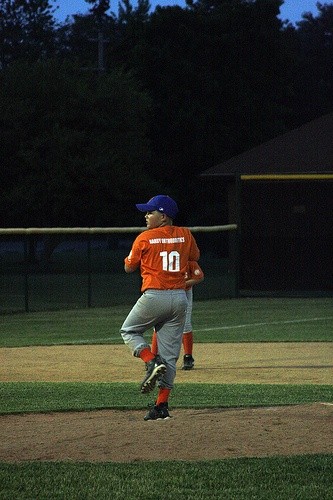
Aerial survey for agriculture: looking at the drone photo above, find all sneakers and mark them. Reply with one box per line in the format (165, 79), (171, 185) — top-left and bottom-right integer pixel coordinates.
(182, 354), (194, 370)
(143, 401), (169, 421)
(140, 355), (166, 394)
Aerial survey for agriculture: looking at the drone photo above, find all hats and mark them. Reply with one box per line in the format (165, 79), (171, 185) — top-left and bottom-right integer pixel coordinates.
(135, 195), (178, 219)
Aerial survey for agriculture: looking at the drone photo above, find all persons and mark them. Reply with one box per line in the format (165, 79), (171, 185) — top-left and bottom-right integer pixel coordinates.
(145, 261), (205, 371)
(119, 194), (201, 421)
